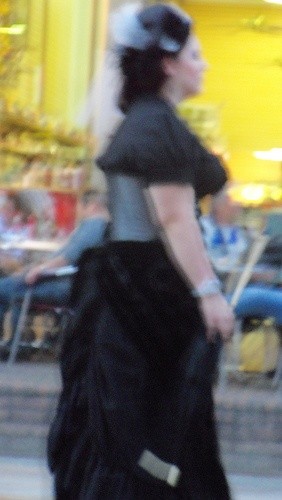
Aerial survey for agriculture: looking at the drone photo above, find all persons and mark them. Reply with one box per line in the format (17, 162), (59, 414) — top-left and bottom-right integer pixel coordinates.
(0, 189), (33, 274)
(199, 188), (243, 259)
(0, 188), (112, 361)
(47, 4), (235, 500)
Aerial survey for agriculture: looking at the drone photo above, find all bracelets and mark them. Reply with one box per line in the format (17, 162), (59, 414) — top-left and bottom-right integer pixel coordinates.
(191, 279), (222, 298)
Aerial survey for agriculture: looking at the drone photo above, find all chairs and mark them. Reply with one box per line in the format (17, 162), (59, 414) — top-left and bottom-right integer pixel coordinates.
(0, 264), (79, 366)
(222, 231), (273, 309)
